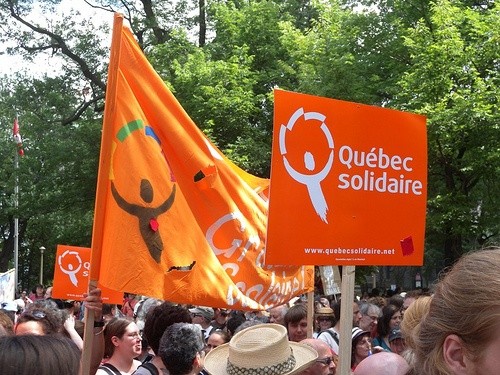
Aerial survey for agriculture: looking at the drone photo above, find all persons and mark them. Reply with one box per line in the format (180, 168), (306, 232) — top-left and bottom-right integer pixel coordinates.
(400, 246), (500, 375)
(0, 278), (436, 375)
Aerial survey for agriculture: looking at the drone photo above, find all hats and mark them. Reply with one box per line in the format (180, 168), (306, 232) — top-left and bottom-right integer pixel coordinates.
(203, 323), (320, 375)
(352, 326), (371, 345)
(314, 308), (337, 322)
(32, 285), (43, 294)
(188, 305), (214, 321)
(389, 327), (406, 341)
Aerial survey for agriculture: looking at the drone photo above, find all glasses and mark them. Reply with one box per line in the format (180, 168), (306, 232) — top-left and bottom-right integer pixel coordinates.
(316, 356), (334, 365)
(317, 316), (336, 322)
(17, 310), (56, 331)
(193, 314), (203, 318)
(365, 314), (380, 321)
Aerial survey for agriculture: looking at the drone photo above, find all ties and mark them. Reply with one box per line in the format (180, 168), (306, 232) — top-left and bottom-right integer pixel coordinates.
(201, 330), (206, 337)
